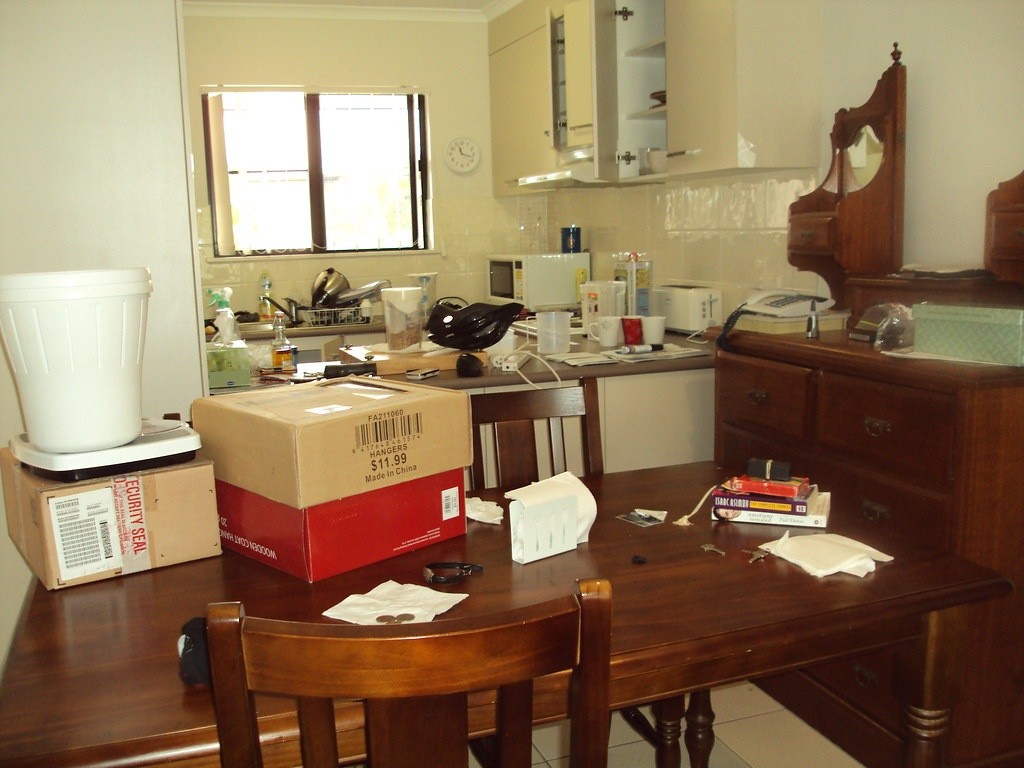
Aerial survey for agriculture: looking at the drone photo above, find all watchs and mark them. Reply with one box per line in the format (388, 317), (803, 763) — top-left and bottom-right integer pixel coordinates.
(422, 562), (483, 585)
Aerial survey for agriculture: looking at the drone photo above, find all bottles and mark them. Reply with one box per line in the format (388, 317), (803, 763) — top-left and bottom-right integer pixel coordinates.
(805, 299), (820, 338)
(271, 311), (298, 374)
(256, 269), (275, 322)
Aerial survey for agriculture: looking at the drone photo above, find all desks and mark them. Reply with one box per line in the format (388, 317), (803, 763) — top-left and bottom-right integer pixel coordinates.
(0, 454), (1015, 768)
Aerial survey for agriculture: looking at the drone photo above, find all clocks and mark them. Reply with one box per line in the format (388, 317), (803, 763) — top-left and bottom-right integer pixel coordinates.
(445, 136), (481, 174)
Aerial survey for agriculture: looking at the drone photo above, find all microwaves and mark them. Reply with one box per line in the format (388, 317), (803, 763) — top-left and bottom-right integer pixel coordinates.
(487, 252), (591, 309)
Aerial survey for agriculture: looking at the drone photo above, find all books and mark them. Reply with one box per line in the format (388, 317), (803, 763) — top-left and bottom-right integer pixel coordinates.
(709, 474), (833, 529)
(735, 308), (852, 334)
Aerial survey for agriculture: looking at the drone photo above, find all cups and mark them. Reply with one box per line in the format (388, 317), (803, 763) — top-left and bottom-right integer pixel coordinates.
(647, 150), (667, 173)
(380, 287), (423, 351)
(561, 226), (582, 253)
(526, 312), (574, 354)
(640, 316), (667, 344)
(588, 316), (621, 347)
(621, 315), (644, 346)
(407, 271), (438, 323)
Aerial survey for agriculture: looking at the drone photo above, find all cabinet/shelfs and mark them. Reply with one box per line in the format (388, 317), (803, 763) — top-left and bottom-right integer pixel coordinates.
(693, 328), (1024, 768)
(486, 1), (821, 199)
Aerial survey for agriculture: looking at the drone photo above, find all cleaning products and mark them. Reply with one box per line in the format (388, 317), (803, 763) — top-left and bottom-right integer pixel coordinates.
(206, 286), (234, 309)
(256, 267), (277, 321)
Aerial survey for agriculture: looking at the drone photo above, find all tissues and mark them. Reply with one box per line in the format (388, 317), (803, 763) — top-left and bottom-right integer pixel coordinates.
(206, 308), (252, 389)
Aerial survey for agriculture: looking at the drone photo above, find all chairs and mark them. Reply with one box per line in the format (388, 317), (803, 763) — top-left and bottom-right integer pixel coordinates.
(466, 376), (605, 491)
(204, 576), (613, 767)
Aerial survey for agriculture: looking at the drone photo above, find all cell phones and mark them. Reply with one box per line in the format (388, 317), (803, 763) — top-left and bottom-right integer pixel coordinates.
(405, 367), (440, 380)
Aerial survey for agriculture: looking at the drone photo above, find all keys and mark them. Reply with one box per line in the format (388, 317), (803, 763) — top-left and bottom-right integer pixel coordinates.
(743, 548), (771, 559)
(701, 543), (726, 555)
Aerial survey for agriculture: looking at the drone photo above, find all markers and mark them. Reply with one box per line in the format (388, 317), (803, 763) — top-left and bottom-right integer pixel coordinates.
(621, 344), (664, 354)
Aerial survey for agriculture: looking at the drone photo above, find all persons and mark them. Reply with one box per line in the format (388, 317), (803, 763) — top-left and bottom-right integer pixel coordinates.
(713, 508), (741, 521)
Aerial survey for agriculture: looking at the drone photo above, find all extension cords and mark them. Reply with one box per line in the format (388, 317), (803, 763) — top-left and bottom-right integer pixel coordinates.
(501, 350), (532, 372)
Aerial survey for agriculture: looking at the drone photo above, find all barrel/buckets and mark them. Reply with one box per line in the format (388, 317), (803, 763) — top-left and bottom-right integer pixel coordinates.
(0, 268), (154, 453)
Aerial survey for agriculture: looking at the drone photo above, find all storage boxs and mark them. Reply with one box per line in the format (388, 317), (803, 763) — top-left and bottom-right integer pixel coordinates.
(0, 445), (223, 593)
(911, 298), (1024, 367)
(191, 375), (473, 509)
(214, 468), (471, 585)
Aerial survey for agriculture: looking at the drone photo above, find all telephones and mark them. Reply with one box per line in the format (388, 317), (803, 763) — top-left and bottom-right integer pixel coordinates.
(738, 288), (836, 319)
